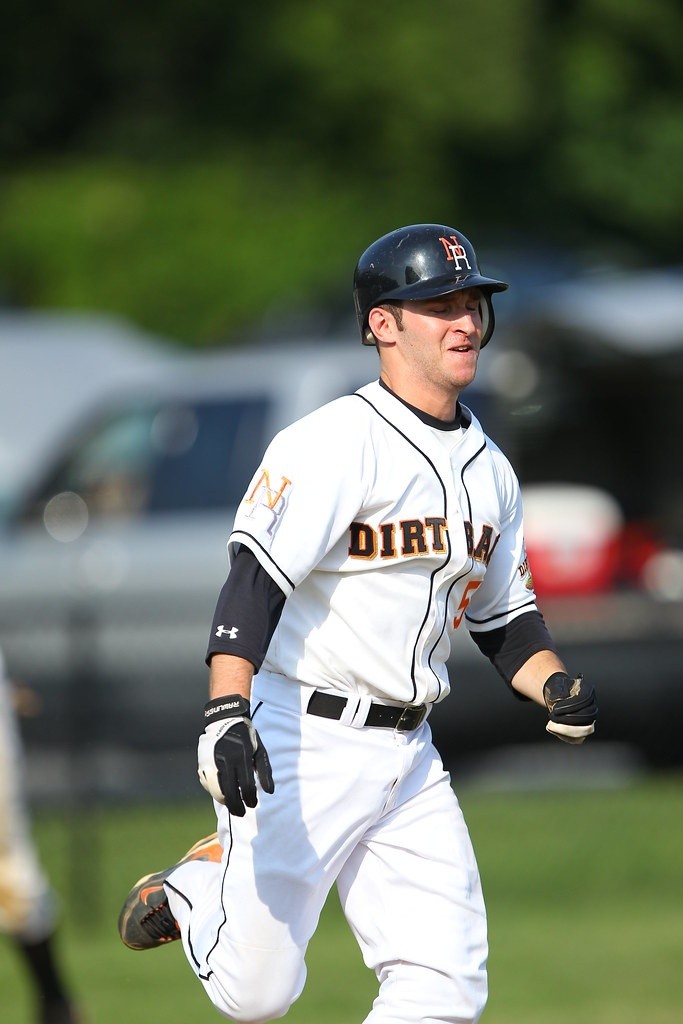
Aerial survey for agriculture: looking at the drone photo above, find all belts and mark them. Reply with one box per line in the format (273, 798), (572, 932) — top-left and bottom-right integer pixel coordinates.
(305, 690), (427, 732)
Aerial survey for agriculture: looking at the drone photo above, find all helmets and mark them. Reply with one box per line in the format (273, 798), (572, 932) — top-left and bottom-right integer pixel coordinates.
(355, 222), (510, 350)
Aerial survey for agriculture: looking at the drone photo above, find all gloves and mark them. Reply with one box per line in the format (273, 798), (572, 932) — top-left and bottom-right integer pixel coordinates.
(542, 670), (599, 745)
(197, 693), (275, 818)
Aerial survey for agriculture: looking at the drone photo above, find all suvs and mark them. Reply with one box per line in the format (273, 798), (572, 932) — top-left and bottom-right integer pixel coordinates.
(0, 309), (679, 794)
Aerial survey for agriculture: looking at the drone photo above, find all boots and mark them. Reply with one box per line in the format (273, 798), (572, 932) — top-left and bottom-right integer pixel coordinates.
(24, 936), (72, 1024)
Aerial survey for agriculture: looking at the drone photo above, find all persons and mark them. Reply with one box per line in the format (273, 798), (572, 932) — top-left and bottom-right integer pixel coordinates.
(116, 224), (597, 1023)
(0, 656), (70, 1024)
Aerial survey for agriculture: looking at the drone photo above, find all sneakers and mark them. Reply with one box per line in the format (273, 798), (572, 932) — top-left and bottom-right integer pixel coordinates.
(116, 831), (224, 953)
